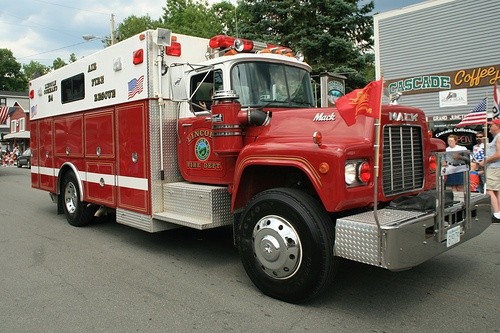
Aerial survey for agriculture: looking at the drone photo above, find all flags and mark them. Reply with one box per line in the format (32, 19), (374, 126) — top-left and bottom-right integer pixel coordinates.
(335, 77), (383, 126)
(0, 106), (20, 125)
(456, 96), (487, 126)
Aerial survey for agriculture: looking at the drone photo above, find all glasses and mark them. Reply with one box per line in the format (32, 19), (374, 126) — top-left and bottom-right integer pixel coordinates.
(476, 136), (484, 138)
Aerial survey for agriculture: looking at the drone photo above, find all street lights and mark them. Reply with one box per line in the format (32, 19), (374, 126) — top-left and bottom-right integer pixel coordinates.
(82, 17), (116, 46)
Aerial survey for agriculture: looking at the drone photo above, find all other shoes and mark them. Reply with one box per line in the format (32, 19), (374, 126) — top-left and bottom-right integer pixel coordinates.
(491, 214), (500, 223)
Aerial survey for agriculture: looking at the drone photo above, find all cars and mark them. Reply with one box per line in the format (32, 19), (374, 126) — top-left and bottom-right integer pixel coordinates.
(17, 148), (31, 169)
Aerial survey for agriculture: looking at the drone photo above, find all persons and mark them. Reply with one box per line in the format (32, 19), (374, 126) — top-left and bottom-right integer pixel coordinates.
(486, 118), (500, 223)
(444, 135), (473, 192)
(0, 141), (22, 168)
(473, 131), (487, 195)
(199, 88), (213, 110)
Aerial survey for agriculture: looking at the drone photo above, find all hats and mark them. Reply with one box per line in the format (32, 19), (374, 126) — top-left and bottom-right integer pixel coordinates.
(487, 119), (500, 124)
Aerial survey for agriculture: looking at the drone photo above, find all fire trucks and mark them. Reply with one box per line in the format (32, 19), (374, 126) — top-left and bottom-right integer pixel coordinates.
(27, 27), (492, 305)
